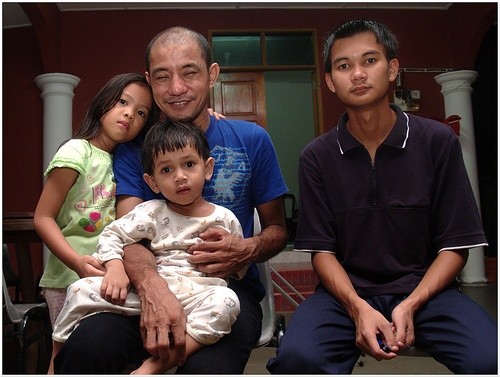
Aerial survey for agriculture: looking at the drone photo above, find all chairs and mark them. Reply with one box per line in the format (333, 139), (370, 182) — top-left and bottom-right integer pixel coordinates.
(250, 208), (286, 349)
(2, 244), (54, 375)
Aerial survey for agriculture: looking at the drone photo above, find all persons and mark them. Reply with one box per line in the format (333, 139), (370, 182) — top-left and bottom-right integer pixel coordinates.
(54, 26), (289, 375)
(34, 73), (227, 375)
(266, 20), (498, 375)
(47, 120), (250, 375)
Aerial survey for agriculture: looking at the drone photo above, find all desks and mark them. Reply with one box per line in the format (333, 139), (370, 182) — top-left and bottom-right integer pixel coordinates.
(2, 219), (42, 303)
